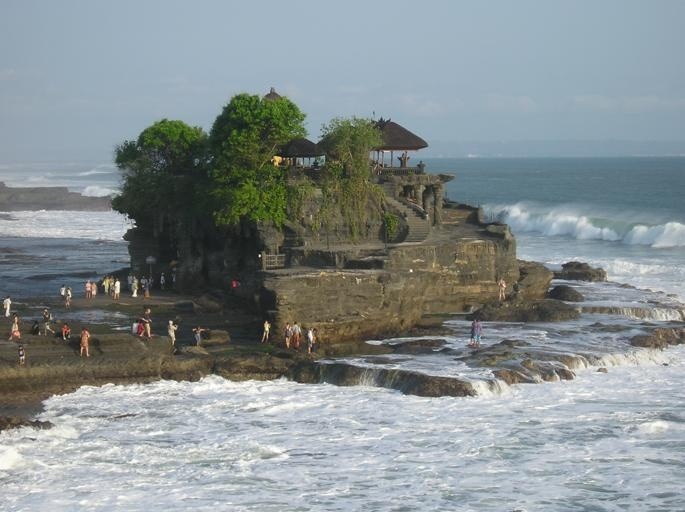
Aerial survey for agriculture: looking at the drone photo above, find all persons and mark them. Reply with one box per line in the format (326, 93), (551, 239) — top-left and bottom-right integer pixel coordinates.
(513, 280), (520, 294)
(3, 296), (209, 366)
(470, 319), (483, 347)
(498, 277), (507, 301)
(58, 260), (177, 306)
(260, 320), (318, 354)
